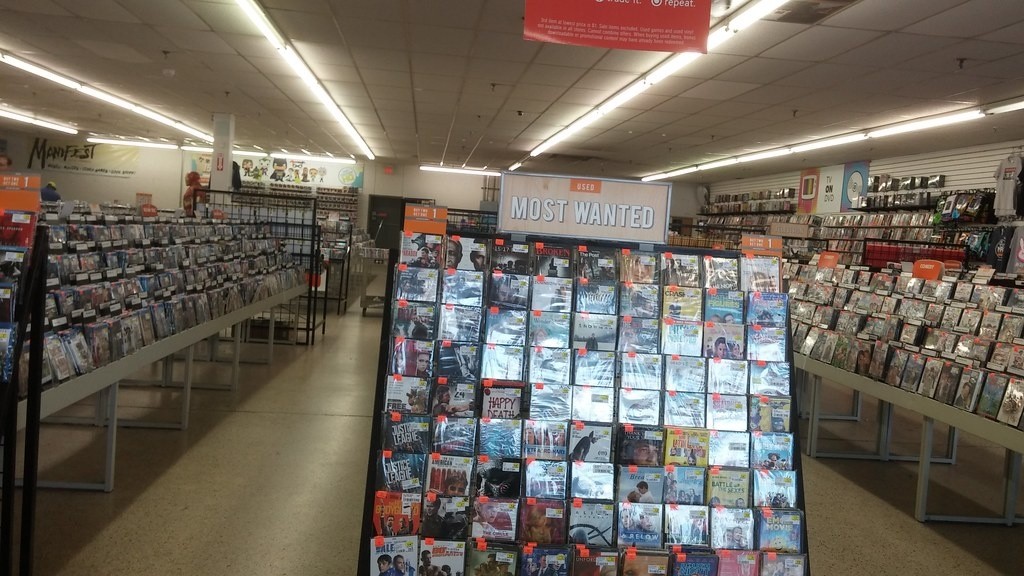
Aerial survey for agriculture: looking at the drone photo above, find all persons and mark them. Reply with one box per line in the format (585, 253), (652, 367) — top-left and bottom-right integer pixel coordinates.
(361, 228), (1024, 576)
(40, 182), (62, 202)
(183, 171), (207, 217)
(0, 155), (13, 172)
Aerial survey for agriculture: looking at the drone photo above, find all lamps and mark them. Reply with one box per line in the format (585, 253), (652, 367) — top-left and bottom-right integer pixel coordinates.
(0, 0), (1024, 188)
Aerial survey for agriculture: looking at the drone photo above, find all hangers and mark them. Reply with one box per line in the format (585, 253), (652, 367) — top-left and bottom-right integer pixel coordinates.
(1008, 145), (1021, 159)
(934, 184), (1014, 246)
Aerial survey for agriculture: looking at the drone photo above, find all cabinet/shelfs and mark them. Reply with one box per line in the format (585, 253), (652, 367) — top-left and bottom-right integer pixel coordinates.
(0, 190), (326, 576)
(823, 203), (938, 252)
(240, 179), (375, 316)
(783, 258), (1024, 520)
(696, 210), (820, 243)
(358, 201), (809, 576)
